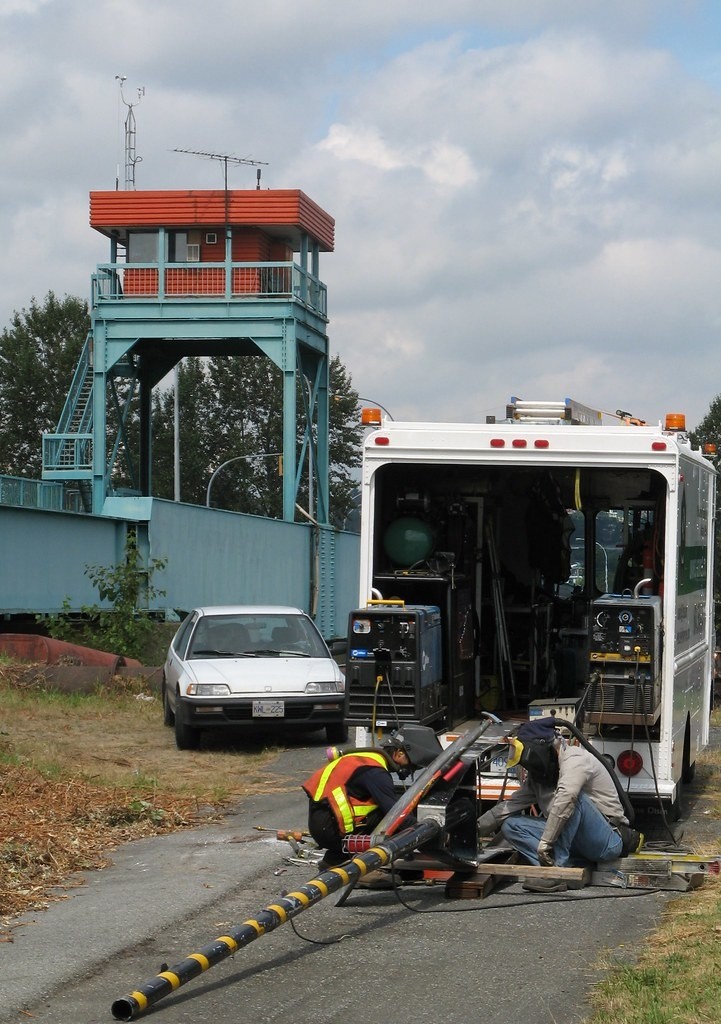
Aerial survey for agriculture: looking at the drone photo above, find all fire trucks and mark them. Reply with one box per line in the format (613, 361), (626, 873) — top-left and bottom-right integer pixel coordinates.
(345, 393), (721, 824)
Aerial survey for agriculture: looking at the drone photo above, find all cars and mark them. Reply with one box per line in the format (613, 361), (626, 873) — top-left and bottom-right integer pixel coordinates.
(159, 605), (353, 752)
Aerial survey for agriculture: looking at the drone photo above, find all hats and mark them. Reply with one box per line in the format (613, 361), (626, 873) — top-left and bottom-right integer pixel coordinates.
(517, 717), (556, 744)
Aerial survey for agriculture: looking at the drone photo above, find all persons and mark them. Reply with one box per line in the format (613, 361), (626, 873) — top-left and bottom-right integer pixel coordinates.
(476, 717), (639, 892)
(300, 724), (445, 889)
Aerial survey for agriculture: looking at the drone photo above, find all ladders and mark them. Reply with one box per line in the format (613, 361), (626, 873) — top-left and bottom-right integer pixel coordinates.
(507, 395), (603, 425)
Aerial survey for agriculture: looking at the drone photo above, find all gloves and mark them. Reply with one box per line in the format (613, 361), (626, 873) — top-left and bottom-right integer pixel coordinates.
(475, 810), (499, 837)
(536, 812), (567, 867)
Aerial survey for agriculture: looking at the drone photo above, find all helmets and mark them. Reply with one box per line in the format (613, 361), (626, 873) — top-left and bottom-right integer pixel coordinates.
(379, 725), (444, 768)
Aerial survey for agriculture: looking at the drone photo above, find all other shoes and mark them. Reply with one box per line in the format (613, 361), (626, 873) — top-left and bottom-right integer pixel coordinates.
(522, 877), (567, 893)
(318, 860), (331, 872)
(354, 868), (402, 889)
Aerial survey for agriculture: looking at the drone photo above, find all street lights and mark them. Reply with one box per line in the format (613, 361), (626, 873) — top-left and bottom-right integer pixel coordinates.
(575, 536), (609, 594)
